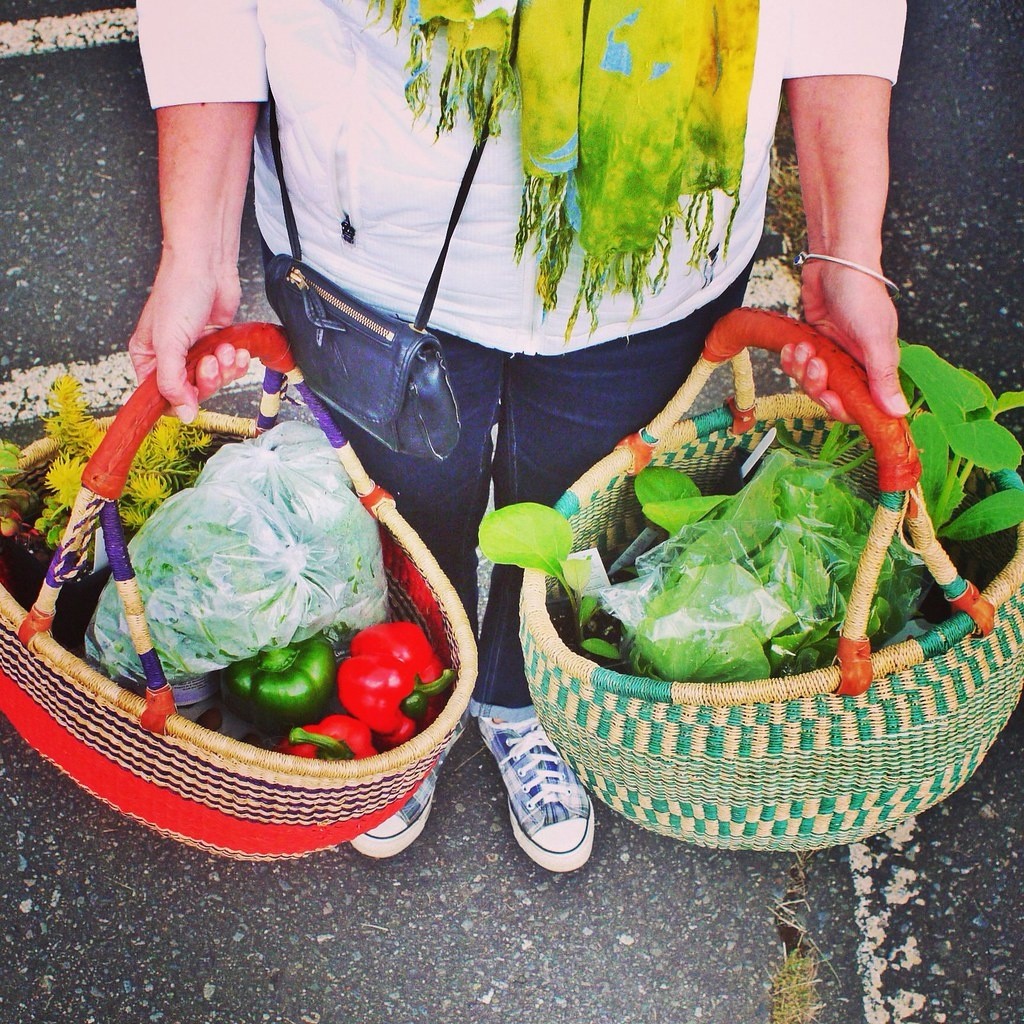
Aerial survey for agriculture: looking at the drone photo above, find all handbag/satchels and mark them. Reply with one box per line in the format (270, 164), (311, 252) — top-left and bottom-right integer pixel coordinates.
(263, 253), (461, 467)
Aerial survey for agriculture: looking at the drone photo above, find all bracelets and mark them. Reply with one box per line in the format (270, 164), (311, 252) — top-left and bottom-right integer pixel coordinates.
(793, 251), (899, 294)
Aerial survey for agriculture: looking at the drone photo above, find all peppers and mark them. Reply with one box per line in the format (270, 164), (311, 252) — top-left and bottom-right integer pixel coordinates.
(220, 620), (456, 762)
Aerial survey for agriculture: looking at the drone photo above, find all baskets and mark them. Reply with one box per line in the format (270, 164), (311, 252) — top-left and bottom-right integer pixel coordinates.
(516, 306), (1024, 853)
(0, 322), (479, 864)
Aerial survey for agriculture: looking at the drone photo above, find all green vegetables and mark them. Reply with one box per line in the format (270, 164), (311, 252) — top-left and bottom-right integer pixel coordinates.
(81, 423), (388, 688)
(477, 336), (1024, 684)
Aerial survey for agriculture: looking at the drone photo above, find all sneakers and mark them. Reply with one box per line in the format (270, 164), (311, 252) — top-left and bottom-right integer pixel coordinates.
(476, 714), (595, 873)
(349, 719), (467, 858)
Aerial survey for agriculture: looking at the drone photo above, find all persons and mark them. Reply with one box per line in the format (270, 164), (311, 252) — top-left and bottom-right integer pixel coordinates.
(129, 0), (914, 876)
